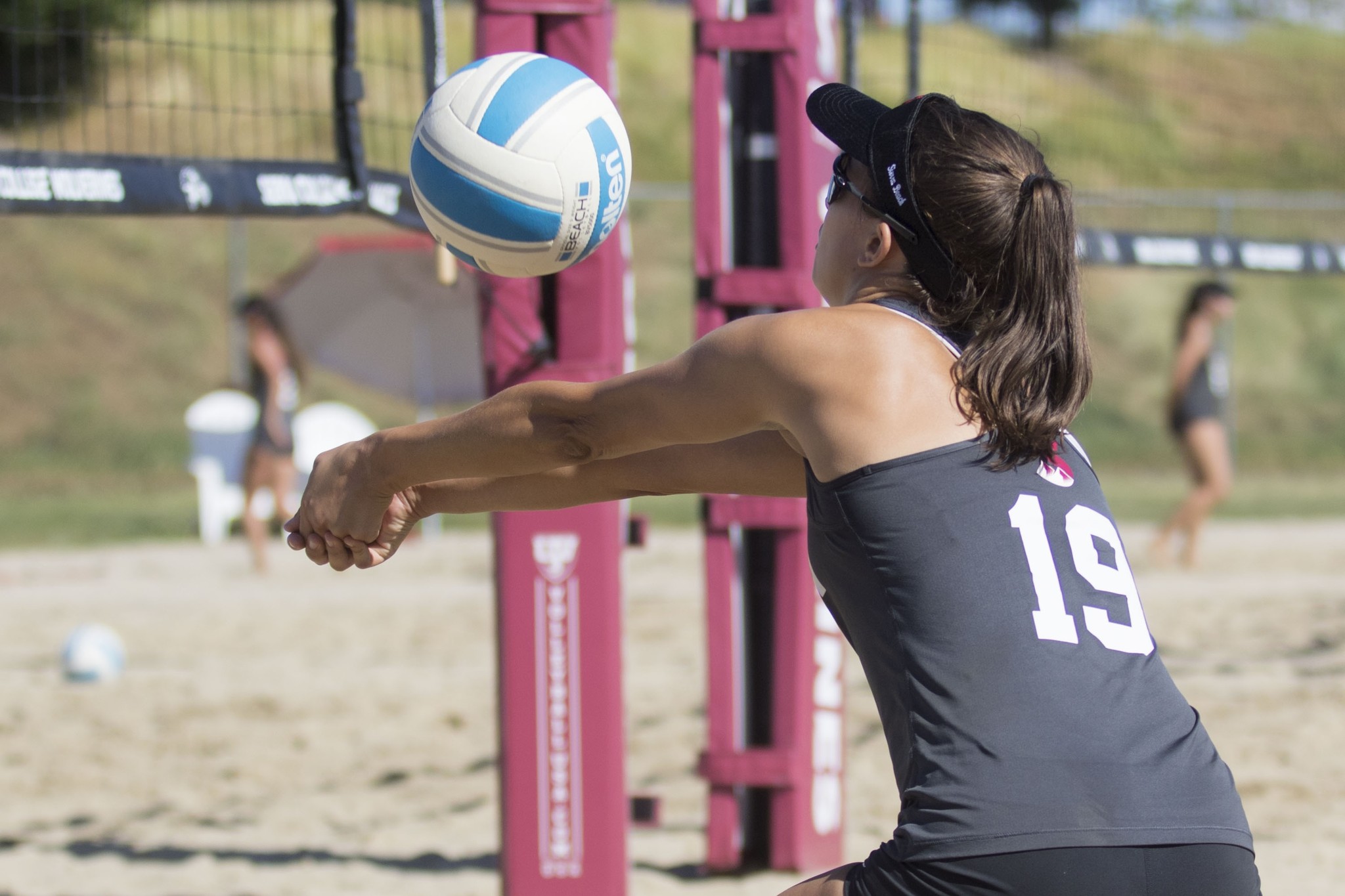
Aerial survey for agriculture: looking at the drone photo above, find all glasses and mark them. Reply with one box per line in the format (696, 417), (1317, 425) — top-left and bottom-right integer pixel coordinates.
(826, 153), (919, 247)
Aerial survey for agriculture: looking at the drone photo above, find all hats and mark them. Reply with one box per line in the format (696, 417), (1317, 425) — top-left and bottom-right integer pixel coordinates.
(806, 82), (980, 306)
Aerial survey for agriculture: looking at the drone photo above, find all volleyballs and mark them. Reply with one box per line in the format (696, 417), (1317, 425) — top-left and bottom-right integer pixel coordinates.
(63, 623), (127, 680)
(409, 51), (632, 276)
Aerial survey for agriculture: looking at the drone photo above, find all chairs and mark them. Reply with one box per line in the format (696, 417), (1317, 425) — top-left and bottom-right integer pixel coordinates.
(179, 389), (396, 554)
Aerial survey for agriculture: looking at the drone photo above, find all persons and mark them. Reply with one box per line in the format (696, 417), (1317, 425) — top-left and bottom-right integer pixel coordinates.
(234, 297), (304, 575)
(279, 84), (1265, 896)
(1147, 281), (1238, 575)
